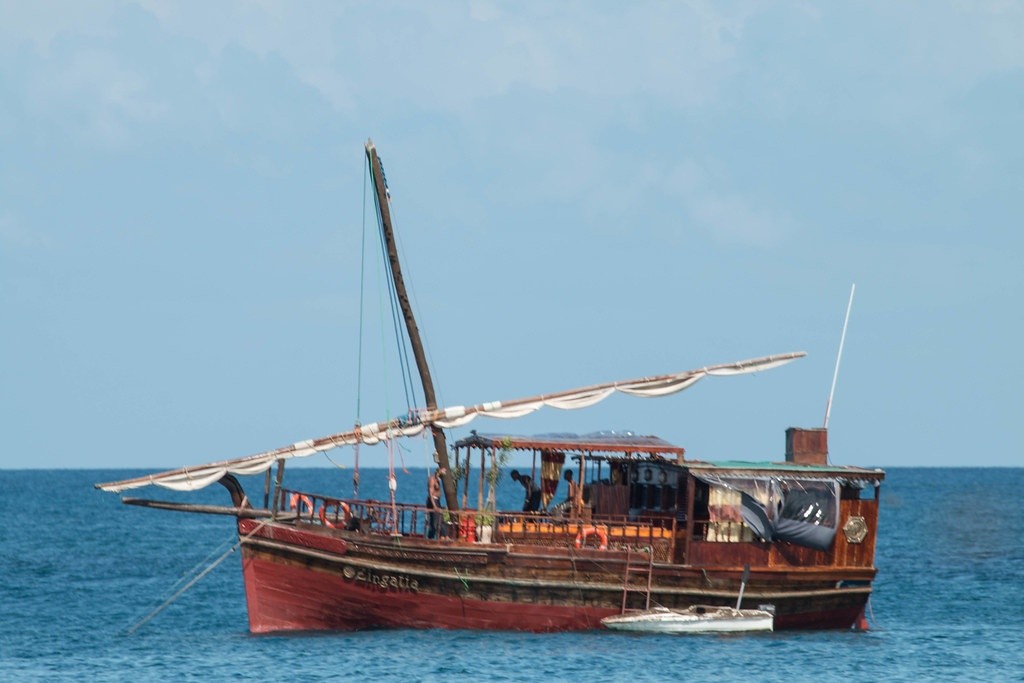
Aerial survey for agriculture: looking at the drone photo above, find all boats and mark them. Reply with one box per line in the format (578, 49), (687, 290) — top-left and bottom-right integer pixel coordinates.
(90, 138), (886, 636)
(599, 604), (773, 634)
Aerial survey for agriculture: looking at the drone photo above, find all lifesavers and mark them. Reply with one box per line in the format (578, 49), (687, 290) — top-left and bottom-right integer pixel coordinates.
(288, 493), (315, 515)
(318, 500), (352, 531)
(573, 527), (610, 551)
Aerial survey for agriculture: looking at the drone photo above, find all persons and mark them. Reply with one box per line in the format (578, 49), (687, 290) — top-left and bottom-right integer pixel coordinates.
(510, 469), (542, 511)
(425, 466), (455, 543)
(551, 469), (582, 516)
